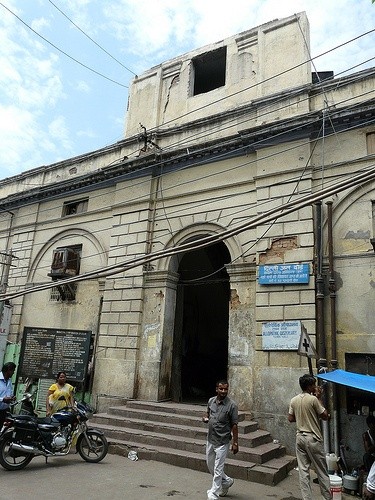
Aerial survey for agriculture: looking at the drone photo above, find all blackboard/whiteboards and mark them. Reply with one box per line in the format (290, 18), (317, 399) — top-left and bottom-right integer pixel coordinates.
(19, 326), (92, 384)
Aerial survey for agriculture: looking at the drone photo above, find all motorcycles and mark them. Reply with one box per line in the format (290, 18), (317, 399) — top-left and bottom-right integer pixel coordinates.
(10, 387), (40, 417)
(0, 386), (110, 472)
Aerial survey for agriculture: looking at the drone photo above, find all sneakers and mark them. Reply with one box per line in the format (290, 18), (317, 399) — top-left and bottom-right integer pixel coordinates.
(218, 478), (234, 497)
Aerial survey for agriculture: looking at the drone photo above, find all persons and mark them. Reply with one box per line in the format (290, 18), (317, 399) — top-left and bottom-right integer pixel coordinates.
(288, 374), (332, 500)
(363, 461), (375, 500)
(46, 372), (77, 454)
(0, 362), (16, 432)
(362, 415), (375, 471)
(202, 380), (239, 499)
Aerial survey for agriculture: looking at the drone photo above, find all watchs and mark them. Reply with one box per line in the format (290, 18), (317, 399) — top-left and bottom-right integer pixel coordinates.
(233, 442), (238, 446)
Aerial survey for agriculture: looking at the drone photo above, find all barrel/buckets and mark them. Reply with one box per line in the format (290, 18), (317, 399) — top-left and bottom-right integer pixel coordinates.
(325, 453), (338, 471)
(327, 475), (342, 500)
